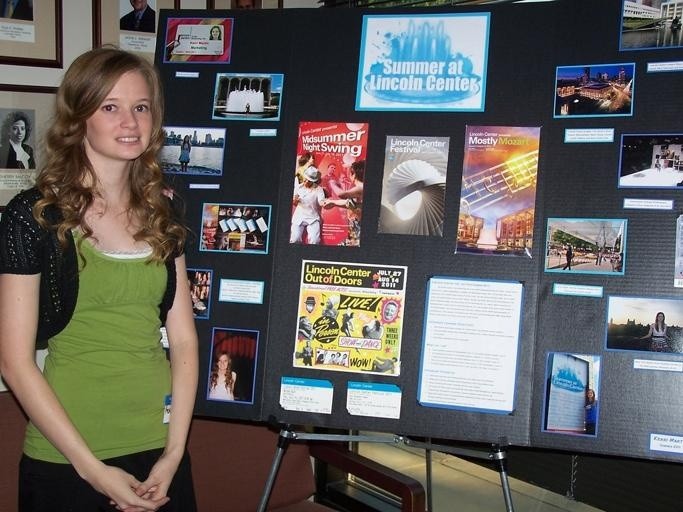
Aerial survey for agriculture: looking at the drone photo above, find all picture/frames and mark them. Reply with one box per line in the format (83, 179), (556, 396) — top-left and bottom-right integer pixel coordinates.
(0, 0), (102, 94)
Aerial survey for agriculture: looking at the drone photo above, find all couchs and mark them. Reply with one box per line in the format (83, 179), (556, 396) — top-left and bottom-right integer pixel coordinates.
(0, 390), (425, 512)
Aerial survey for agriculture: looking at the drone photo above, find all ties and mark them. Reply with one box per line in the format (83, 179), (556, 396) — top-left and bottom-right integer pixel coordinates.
(134, 13), (141, 27)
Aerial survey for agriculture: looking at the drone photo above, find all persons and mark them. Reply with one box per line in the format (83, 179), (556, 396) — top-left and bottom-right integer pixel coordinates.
(290, 152), (366, 246)
(236, 0), (256, 9)
(244, 102), (250, 118)
(562, 247), (575, 270)
(299, 296), (397, 373)
(210, 350), (237, 402)
(0, 112), (34, 170)
(640, 312), (673, 352)
(584, 389), (598, 435)
(120, 0), (156, 33)
(179, 135), (191, 173)
(0, 47), (200, 511)
(209, 26), (222, 40)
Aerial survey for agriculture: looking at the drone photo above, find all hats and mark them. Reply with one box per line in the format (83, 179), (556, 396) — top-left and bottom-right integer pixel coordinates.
(303, 166), (319, 182)
